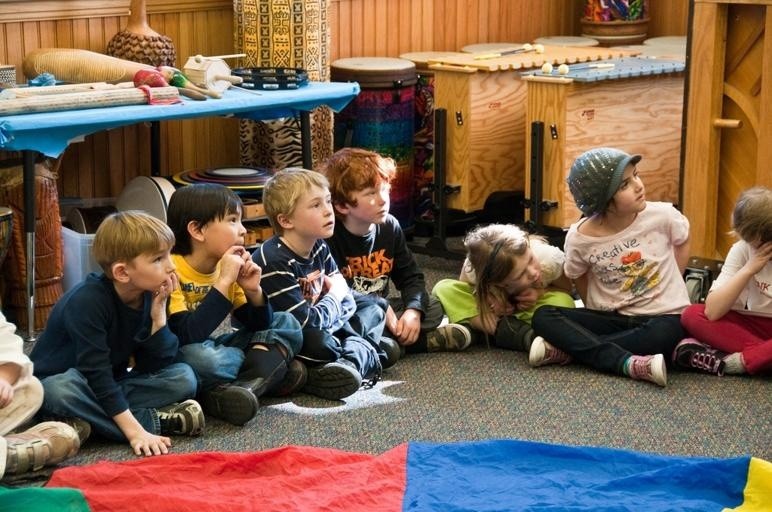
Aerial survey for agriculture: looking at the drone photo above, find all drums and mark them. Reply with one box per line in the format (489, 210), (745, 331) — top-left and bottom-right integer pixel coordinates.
(331, 51), (482, 240)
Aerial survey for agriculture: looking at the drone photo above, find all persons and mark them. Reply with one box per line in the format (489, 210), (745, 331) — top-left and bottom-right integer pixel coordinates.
(528, 148), (692, 388)
(164, 183), (309, 426)
(679, 185), (772, 377)
(0, 311), (81, 480)
(431, 221), (576, 367)
(321, 148), (472, 367)
(29, 209), (207, 458)
(250, 168), (386, 400)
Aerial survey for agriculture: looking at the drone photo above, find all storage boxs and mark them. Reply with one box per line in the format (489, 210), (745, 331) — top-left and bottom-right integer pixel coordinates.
(57, 198), (125, 294)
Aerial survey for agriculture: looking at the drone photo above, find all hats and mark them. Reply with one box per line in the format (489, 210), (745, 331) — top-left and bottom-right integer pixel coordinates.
(567, 146), (642, 213)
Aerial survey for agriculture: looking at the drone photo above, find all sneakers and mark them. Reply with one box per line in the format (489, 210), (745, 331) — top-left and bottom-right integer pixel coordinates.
(308, 359), (364, 401)
(670, 337), (725, 375)
(528, 336), (568, 368)
(37, 413), (91, 448)
(157, 397), (205, 440)
(628, 352), (667, 390)
(378, 334), (401, 371)
(201, 383), (260, 425)
(4, 422), (79, 478)
(272, 358), (308, 397)
(425, 323), (472, 351)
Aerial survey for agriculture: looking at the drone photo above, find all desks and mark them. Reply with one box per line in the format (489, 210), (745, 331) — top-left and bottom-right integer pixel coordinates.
(0, 78), (361, 343)
(408, 66), (526, 260)
(522, 71), (685, 237)
